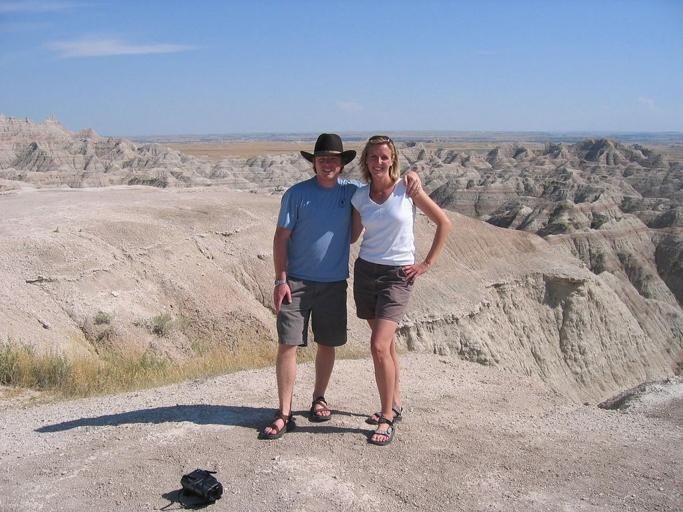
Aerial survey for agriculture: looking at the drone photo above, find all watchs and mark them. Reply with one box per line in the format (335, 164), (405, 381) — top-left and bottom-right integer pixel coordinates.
(274, 280), (286, 286)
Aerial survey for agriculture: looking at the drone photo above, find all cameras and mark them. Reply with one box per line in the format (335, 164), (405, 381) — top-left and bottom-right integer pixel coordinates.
(181, 469), (223, 504)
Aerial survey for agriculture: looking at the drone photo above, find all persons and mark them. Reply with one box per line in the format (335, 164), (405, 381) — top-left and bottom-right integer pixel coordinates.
(264, 134), (422, 438)
(350, 135), (450, 445)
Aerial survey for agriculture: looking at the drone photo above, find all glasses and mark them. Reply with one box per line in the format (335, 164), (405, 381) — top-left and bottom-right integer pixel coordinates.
(369, 135), (389, 140)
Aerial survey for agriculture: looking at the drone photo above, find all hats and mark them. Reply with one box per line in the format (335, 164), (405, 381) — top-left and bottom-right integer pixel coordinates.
(300, 134), (356, 165)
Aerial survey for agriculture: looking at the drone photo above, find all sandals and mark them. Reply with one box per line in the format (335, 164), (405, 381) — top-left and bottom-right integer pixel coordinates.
(311, 396), (332, 420)
(370, 414), (395, 446)
(367, 407), (402, 425)
(261, 411), (295, 439)
